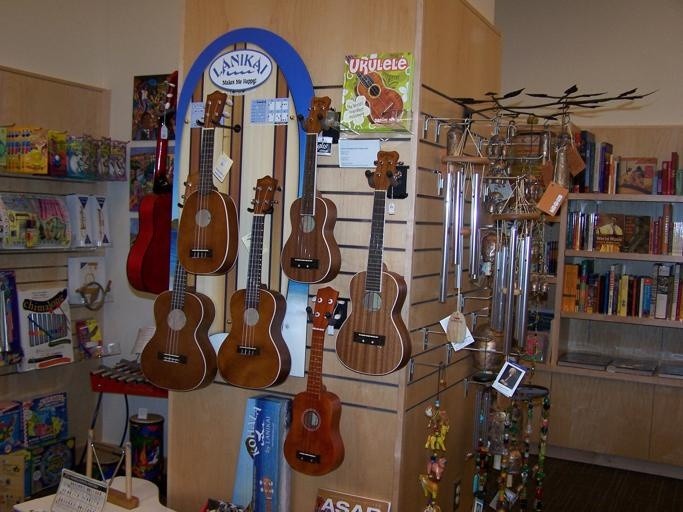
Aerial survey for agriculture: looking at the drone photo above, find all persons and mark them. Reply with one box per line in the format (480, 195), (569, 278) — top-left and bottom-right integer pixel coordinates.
(498, 366), (519, 389)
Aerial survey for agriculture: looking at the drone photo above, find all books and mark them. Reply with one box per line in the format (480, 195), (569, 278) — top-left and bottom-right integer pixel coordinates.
(556, 348), (616, 372)
(560, 129), (682, 327)
(606, 353), (658, 377)
(655, 350), (682, 381)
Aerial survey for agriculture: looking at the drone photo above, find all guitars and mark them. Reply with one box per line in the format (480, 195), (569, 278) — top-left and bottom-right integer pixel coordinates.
(335, 151), (412, 375)
(279, 96), (342, 284)
(284, 287), (344, 476)
(347, 56), (403, 125)
(138, 180), (218, 392)
(217, 175), (291, 390)
(127, 72), (178, 297)
(176, 89), (240, 277)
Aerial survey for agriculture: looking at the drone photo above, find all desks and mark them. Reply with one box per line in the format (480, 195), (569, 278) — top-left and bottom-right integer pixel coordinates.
(8, 470), (181, 512)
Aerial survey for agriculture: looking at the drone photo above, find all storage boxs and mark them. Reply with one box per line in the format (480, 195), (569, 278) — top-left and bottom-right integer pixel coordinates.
(224, 394), (291, 512)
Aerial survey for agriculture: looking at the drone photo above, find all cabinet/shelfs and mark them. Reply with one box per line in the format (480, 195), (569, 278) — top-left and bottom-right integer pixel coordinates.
(545, 184), (680, 483)
(482, 117), (564, 467)
(1, 165), (121, 382)
